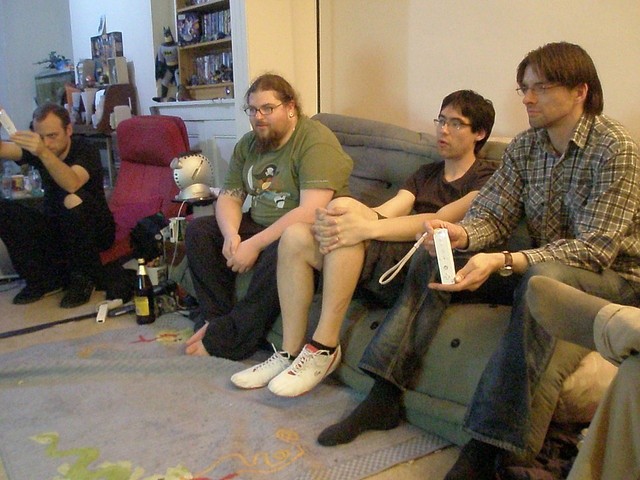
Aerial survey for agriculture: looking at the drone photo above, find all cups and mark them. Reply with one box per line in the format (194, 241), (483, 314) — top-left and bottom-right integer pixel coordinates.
(28, 169), (42, 193)
(169, 217), (186, 244)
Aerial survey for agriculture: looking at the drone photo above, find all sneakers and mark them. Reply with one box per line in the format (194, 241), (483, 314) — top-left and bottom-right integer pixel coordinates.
(231, 350), (295, 389)
(14, 282), (67, 304)
(267, 342), (342, 397)
(60, 282), (94, 308)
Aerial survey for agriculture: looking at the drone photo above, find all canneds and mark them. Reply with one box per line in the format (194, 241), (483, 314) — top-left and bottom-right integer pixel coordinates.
(11, 174), (24, 190)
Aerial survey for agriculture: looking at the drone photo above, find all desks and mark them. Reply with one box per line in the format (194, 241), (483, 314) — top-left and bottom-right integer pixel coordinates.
(71, 125), (114, 189)
(170, 197), (219, 217)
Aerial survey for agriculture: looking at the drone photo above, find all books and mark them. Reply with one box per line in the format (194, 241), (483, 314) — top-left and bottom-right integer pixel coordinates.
(205, 10), (231, 42)
(195, 52), (233, 84)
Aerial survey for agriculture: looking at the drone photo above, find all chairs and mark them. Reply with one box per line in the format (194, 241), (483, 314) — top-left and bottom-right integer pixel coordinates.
(94, 114), (194, 300)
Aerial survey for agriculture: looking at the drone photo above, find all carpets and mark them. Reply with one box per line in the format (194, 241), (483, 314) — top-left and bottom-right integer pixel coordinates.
(0, 305), (454, 480)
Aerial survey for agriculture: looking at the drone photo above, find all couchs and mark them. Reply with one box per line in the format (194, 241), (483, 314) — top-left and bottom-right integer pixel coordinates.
(167, 112), (590, 468)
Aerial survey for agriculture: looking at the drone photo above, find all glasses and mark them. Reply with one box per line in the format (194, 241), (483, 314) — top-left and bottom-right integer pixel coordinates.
(434, 119), (473, 130)
(515, 84), (563, 96)
(244, 103), (282, 116)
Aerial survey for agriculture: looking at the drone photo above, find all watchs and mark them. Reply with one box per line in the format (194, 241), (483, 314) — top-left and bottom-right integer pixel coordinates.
(499, 250), (514, 277)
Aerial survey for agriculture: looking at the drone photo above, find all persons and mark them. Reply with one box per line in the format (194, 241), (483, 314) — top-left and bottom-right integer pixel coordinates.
(231, 89), (498, 398)
(184, 74), (353, 360)
(0, 104), (115, 308)
(152, 25), (182, 102)
(318, 41), (640, 480)
(524, 276), (640, 480)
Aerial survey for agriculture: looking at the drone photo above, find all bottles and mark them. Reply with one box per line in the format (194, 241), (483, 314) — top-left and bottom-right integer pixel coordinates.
(134, 258), (155, 325)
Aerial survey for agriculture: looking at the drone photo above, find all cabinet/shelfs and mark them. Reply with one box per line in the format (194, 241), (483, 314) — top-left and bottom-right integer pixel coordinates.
(151, 0), (233, 103)
(161, 108), (235, 187)
